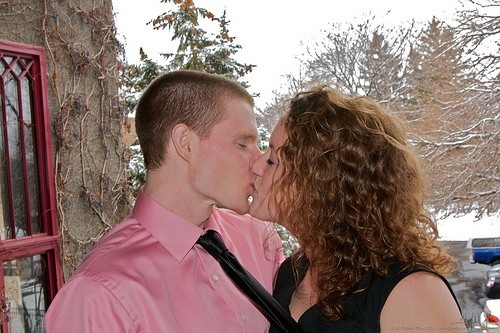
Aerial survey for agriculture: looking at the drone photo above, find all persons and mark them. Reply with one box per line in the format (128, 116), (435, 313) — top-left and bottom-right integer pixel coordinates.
(43, 69), (288, 333)
(249, 87), (469, 333)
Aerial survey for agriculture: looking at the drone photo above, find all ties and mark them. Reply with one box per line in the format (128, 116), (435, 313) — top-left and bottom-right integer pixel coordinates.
(195, 230), (306, 333)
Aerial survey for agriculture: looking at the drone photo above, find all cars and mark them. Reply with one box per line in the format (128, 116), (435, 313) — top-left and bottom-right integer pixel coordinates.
(465, 236), (500, 333)
